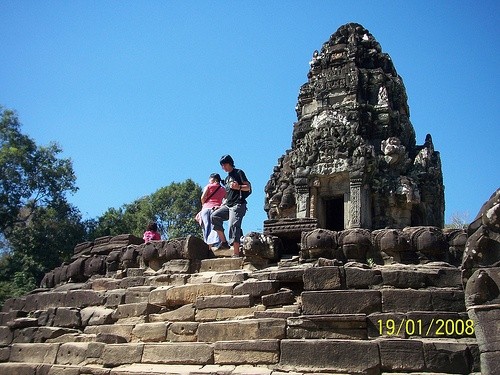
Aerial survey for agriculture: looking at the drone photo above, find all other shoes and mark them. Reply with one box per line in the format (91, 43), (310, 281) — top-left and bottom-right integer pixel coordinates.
(232, 254), (239, 258)
(215, 241), (231, 252)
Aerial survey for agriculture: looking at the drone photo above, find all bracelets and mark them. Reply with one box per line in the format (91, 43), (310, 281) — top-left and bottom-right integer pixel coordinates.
(239, 185), (242, 191)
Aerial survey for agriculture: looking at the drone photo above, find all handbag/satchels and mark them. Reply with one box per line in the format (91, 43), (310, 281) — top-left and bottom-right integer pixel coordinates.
(195, 211), (203, 226)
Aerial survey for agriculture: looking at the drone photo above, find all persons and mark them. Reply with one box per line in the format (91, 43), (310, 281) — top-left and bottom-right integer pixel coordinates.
(210, 155), (252, 258)
(142, 222), (161, 243)
(201, 173), (226, 250)
